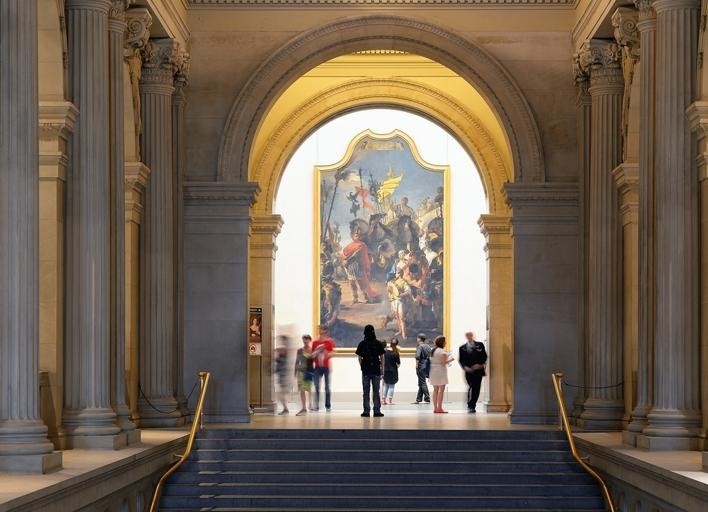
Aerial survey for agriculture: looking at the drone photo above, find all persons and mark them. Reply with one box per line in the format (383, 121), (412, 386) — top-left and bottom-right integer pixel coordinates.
(429, 336), (454, 414)
(381, 338), (401, 404)
(355, 324), (386, 417)
(458, 331), (488, 413)
(320, 186), (443, 341)
(293, 334), (313, 417)
(273, 335), (292, 415)
(410, 333), (432, 404)
(310, 324), (336, 413)
(249, 317), (261, 337)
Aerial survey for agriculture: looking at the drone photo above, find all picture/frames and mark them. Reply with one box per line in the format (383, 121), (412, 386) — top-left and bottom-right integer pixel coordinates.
(311, 128), (452, 359)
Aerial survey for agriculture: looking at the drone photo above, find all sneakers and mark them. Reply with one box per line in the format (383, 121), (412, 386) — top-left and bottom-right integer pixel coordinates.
(383, 402), (396, 404)
(296, 410), (307, 415)
(374, 413), (384, 416)
(419, 401), (430, 404)
(411, 401), (422, 404)
(470, 409), (476, 412)
(361, 413), (370, 417)
(434, 410), (448, 413)
(278, 409), (289, 415)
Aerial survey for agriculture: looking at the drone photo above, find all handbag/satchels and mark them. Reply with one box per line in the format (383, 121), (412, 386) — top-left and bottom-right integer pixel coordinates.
(418, 356), (429, 377)
(304, 371), (312, 380)
(362, 352), (379, 377)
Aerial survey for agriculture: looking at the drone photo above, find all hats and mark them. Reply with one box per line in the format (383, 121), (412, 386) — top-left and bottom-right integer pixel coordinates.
(364, 325), (374, 334)
(418, 333), (426, 339)
